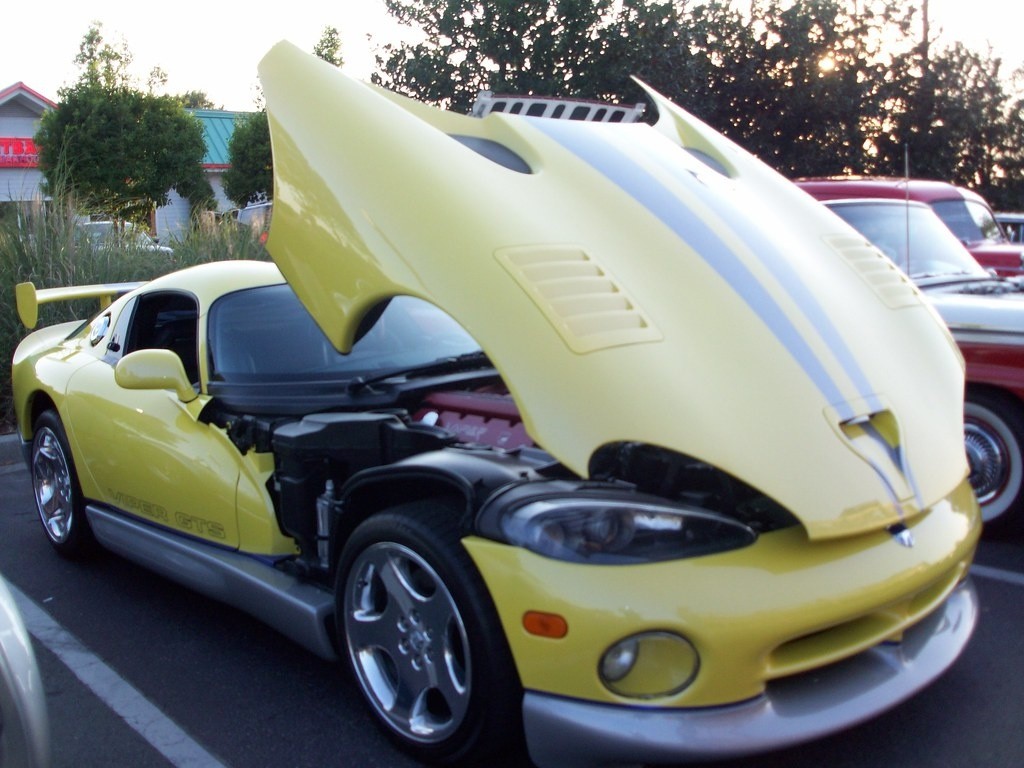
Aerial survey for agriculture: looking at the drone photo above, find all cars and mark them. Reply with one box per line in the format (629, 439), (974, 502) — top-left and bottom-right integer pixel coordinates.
(14, 41), (982, 766)
(788, 176), (1023, 293)
(71, 221), (175, 262)
(818, 198), (1024, 546)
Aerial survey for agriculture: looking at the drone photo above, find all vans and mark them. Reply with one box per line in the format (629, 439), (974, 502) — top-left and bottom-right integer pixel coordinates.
(236, 201), (274, 246)
